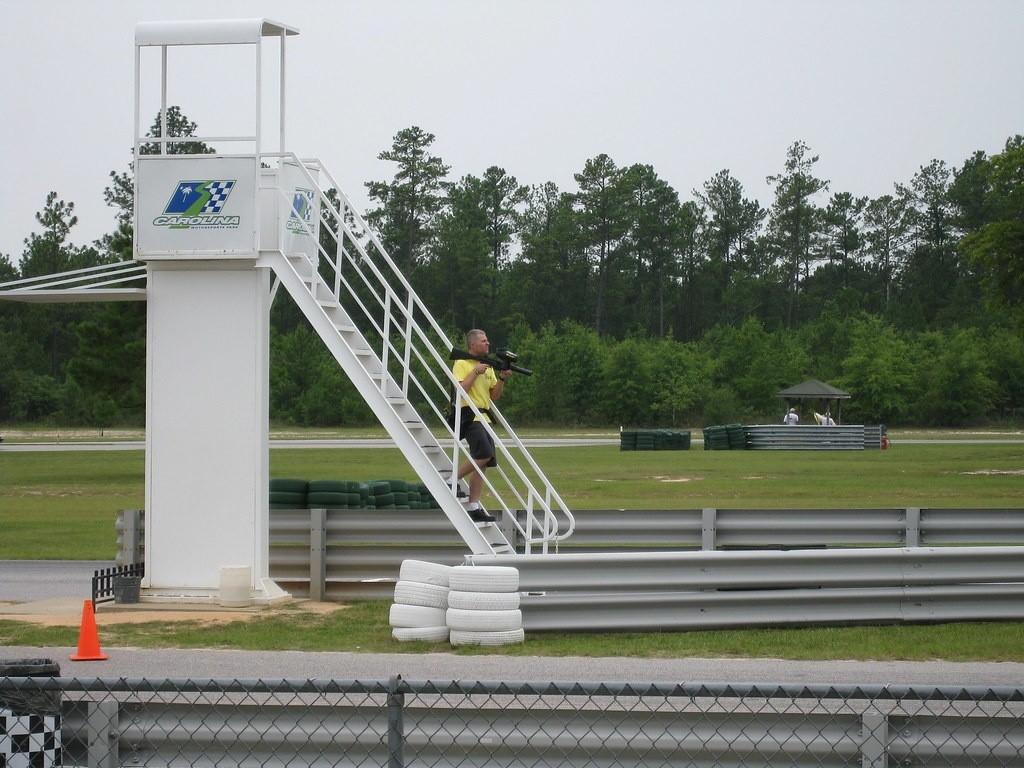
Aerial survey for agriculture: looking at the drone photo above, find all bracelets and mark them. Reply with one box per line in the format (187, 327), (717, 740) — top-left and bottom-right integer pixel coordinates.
(499, 376), (505, 381)
(473, 368), (479, 374)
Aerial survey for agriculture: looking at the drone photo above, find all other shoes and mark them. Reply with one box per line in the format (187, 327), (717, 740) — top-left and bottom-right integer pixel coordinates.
(449, 484), (466, 498)
(467, 509), (496, 522)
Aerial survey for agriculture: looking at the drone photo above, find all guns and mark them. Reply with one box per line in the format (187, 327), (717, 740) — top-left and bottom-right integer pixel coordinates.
(448, 347), (534, 381)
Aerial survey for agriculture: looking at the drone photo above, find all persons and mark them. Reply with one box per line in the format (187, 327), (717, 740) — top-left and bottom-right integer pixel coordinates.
(822, 412), (836, 425)
(445, 329), (512, 521)
(783, 408), (798, 425)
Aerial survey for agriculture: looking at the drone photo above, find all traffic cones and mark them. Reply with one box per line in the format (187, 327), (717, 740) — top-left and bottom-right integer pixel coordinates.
(68, 598), (110, 661)
